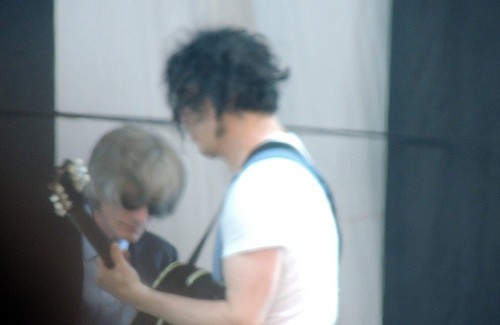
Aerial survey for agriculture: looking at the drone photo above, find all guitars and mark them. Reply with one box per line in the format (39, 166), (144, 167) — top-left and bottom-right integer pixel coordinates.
(34, 160), (224, 325)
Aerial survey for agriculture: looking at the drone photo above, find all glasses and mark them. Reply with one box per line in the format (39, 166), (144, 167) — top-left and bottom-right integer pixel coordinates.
(121, 188), (167, 217)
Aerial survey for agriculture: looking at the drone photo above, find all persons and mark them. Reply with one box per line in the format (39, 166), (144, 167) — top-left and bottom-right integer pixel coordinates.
(4, 127), (189, 325)
(93, 22), (342, 325)
